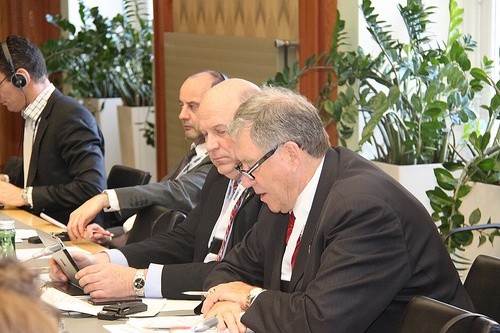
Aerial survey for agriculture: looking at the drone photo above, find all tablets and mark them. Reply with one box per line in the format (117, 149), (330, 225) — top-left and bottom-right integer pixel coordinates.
(36, 228), (86, 290)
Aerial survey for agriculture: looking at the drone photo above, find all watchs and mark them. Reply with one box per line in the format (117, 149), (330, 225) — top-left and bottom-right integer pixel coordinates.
(133, 269), (146, 297)
(246, 288), (263, 304)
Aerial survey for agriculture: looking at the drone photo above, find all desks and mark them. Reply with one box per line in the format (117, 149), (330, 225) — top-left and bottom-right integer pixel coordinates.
(0, 202), (224, 333)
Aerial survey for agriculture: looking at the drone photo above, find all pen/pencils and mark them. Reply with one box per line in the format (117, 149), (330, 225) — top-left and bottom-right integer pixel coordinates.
(84, 228), (114, 237)
(182, 290), (210, 295)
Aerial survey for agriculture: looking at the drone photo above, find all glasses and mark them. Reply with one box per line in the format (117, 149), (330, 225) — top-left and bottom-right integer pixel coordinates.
(234, 140), (301, 180)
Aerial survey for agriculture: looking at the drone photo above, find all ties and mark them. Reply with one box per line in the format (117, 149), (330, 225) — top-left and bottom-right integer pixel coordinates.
(215, 181), (241, 262)
(285, 211), (300, 270)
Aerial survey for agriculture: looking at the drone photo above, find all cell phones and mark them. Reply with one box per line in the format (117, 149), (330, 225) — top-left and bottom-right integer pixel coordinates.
(89, 295), (142, 305)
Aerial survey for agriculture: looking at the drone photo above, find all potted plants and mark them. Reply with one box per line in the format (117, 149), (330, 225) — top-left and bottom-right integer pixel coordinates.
(309, 0), (485, 236)
(430, 50), (500, 284)
(69, 0), (160, 184)
(39, 2), (125, 180)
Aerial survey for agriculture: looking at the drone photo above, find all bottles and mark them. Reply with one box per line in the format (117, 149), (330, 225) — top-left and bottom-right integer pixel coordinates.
(0, 219), (16, 258)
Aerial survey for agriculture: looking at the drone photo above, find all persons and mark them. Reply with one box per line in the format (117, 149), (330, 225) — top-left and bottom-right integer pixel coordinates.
(195, 87), (474, 333)
(67, 71), (227, 247)
(0, 34), (106, 224)
(49, 77), (263, 299)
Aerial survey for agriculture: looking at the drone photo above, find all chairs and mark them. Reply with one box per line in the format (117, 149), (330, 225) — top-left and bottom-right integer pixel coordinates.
(400, 255), (500, 333)
(7, 158), (187, 246)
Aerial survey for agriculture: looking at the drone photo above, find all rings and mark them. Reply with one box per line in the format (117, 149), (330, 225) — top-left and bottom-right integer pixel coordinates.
(214, 314), (217, 317)
(209, 289), (215, 293)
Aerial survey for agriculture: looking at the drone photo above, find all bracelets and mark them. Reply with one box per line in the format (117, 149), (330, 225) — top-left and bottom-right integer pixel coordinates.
(23, 189), (28, 202)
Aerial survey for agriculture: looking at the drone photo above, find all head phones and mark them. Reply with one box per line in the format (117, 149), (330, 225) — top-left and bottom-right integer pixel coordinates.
(1, 40), (27, 88)
